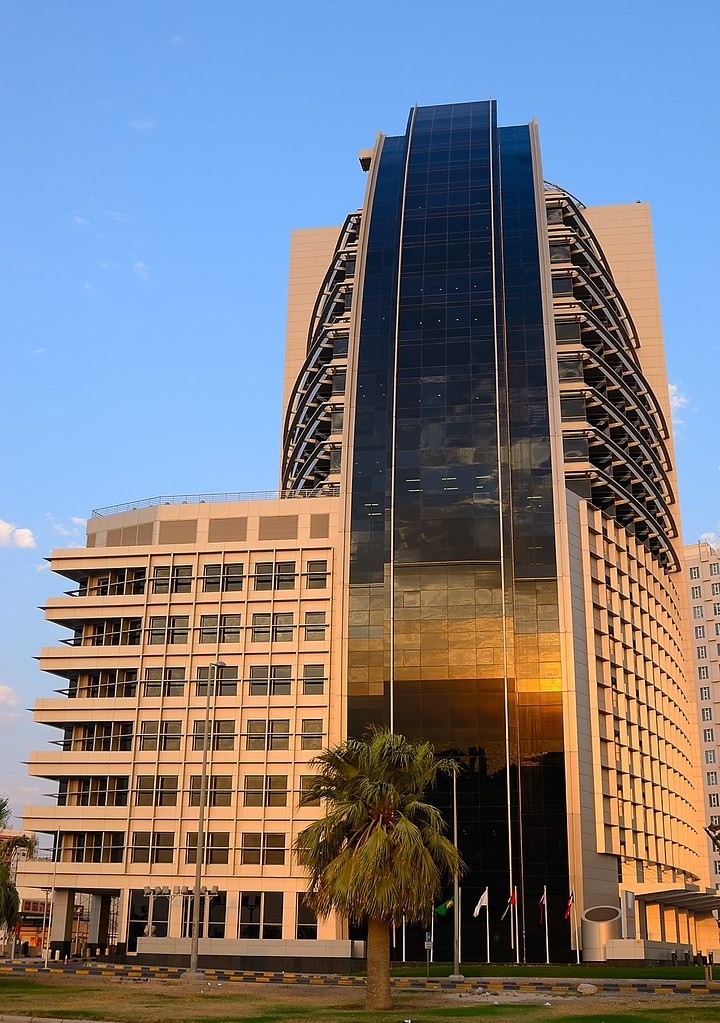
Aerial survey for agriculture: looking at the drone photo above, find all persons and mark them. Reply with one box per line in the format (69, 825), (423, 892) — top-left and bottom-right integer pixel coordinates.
(22, 941), (29, 955)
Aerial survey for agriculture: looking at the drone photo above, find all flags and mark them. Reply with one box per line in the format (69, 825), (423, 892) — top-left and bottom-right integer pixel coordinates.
(539, 895), (545, 924)
(565, 892), (574, 919)
(507, 891), (516, 906)
(435, 897), (454, 915)
(473, 891), (487, 917)
